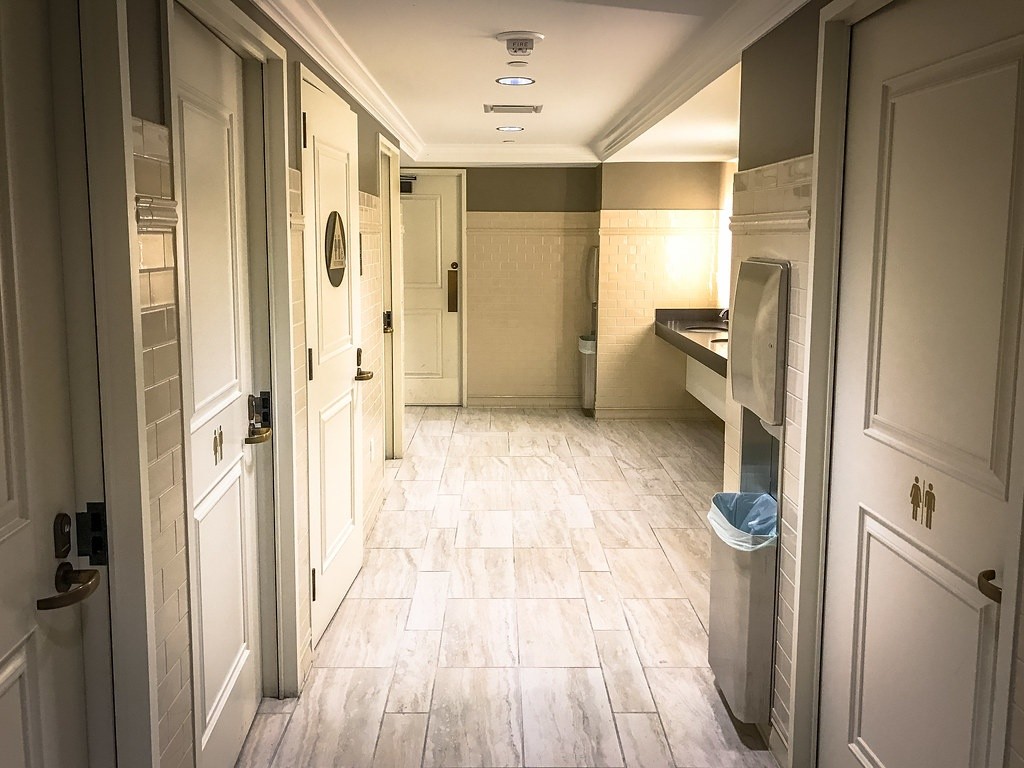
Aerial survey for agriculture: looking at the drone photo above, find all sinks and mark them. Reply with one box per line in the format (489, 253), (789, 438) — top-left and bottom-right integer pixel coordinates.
(712, 339), (728, 343)
(684, 326), (728, 333)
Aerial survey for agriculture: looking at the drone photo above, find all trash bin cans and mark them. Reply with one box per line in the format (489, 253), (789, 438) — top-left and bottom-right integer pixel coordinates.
(709, 490), (777, 723)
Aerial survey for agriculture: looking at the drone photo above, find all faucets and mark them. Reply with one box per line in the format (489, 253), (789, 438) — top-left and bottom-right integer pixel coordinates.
(719, 308), (730, 317)
